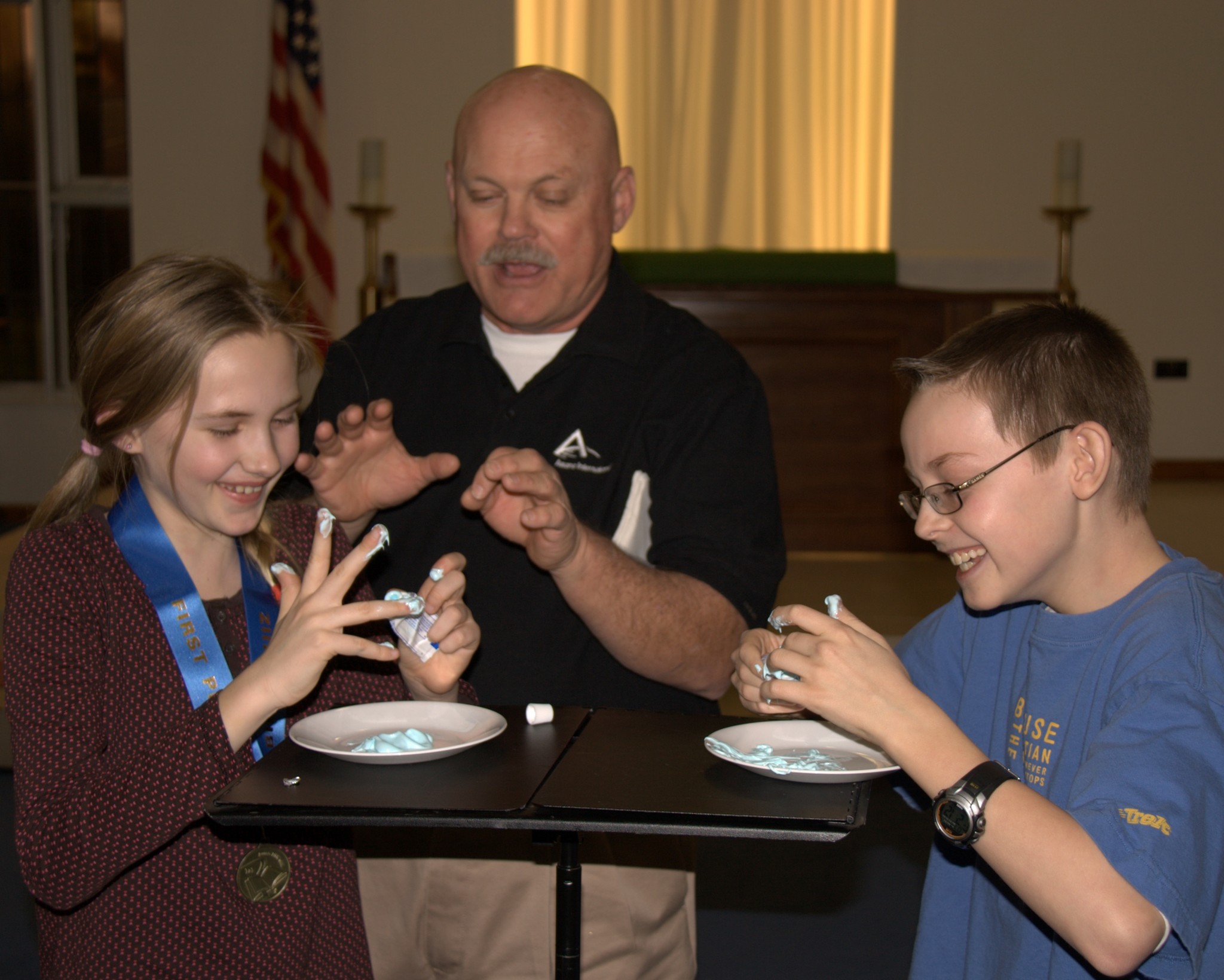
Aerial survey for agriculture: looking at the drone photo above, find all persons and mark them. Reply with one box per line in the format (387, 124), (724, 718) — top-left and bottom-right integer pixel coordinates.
(1, 253), (480, 980)
(728, 296), (1224, 980)
(258, 66), (788, 980)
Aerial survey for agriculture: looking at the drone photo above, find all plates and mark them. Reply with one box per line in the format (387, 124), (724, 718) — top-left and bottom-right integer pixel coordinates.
(288, 699), (508, 765)
(703, 719), (901, 784)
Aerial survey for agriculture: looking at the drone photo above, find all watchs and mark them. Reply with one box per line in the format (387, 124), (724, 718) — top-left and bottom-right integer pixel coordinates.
(932, 760), (1021, 849)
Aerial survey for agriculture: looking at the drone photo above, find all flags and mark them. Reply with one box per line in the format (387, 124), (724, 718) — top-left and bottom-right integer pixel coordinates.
(265, 0), (338, 374)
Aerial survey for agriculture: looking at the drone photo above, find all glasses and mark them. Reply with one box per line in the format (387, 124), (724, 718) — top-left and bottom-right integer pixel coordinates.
(898, 424), (1114, 520)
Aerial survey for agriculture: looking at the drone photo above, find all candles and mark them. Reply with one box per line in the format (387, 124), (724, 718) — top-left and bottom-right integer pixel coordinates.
(359, 138), (387, 202)
(1057, 137), (1080, 206)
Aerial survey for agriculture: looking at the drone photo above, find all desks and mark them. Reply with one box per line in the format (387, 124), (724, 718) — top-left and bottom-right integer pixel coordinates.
(664, 290), (986, 552)
(201, 701), (867, 980)
